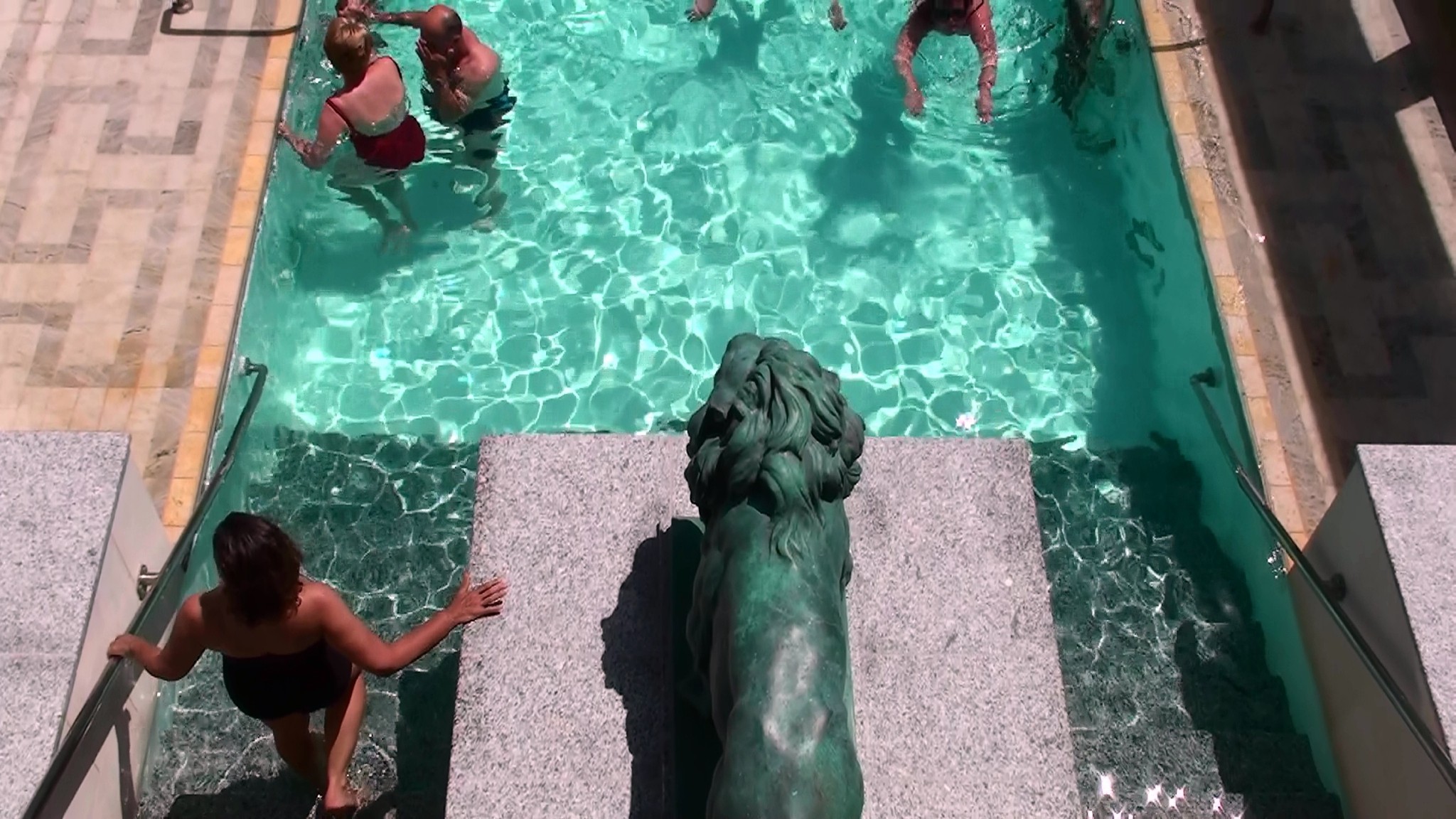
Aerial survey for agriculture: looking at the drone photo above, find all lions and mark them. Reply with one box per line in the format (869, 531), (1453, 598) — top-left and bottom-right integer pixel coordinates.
(682, 331), (866, 819)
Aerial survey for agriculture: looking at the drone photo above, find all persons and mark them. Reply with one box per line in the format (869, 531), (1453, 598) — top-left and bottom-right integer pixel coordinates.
(105, 511), (507, 814)
(894, 0), (997, 121)
(276, 11), (425, 262)
(683, 0), (847, 31)
(340, 6), (518, 208)
